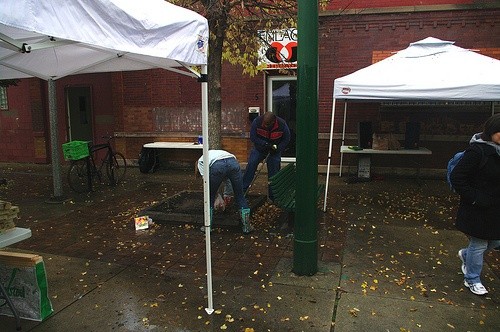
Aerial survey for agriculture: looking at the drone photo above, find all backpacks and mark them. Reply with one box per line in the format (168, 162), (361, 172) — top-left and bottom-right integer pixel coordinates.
(447, 143), (490, 191)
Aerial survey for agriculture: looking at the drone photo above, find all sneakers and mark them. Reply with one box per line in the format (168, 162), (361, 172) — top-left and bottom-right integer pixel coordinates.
(464, 278), (489, 295)
(458, 249), (466, 274)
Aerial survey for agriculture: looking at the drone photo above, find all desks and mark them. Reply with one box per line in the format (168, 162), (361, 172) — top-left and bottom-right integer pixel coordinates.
(338, 145), (432, 183)
(140, 141), (218, 179)
(0, 226), (32, 330)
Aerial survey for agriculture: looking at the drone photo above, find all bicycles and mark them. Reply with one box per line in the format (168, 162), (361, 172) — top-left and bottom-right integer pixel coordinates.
(67, 134), (127, 193)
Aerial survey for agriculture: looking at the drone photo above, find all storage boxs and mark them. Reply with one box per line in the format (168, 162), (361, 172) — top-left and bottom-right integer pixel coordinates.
(1, 251), (54, 322)
(62, 140), (90, 162)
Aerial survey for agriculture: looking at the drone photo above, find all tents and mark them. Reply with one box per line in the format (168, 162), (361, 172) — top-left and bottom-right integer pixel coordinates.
(0, 1), (215, 318)
(319, 34), (500, 214)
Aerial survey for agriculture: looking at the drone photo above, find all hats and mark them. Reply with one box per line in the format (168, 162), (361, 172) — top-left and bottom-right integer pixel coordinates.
(483, 112), (500, 135)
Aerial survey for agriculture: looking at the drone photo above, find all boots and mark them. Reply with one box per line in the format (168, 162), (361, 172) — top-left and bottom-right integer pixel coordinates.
(239, 207), (254, 234)
(200, 207), (214, 233)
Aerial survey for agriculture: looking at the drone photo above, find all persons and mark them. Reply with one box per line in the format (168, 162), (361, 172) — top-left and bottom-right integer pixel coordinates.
(239, 112), (291, 204)
(196, 149), (251, 233)
(447, 113), (500, 295)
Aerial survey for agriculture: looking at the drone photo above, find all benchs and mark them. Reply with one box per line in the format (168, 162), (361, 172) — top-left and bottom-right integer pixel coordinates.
(267, 162), (326, 229)
